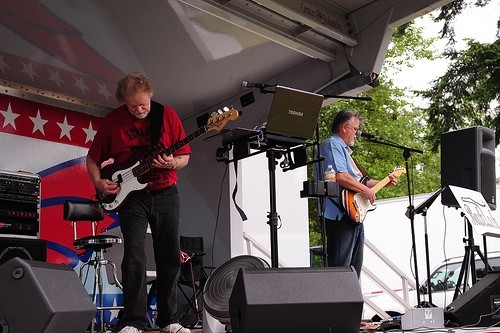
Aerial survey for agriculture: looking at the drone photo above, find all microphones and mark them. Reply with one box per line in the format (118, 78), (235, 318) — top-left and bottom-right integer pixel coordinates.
(357, 131), (383, 139)
(241, 80), (277, 89)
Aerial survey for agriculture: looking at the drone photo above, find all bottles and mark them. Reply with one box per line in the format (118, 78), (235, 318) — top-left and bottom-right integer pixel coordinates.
(325, 164), (336, 182)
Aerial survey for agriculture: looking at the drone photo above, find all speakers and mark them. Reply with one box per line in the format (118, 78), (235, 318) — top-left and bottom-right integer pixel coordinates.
(228, 266), (364, 333)
(444, 271), (500, 326)
(0, 257), (97, 333)
(441, 126), (496, 210)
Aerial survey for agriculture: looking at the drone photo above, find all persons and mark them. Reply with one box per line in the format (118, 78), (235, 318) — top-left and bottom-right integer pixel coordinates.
(313, 108), (398, 280)
(85, 74), (193, 332)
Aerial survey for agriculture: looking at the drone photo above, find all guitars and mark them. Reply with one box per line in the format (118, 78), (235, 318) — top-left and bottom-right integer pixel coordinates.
(340, 165), (408, 225)
(94, 107), (239, 214)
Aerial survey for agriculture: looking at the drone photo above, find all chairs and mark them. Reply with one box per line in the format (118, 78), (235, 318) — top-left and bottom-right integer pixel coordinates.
(62, 200), (126, 333)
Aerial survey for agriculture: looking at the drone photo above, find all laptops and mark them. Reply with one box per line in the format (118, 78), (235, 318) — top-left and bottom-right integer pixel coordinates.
(256, 86), (324, 140)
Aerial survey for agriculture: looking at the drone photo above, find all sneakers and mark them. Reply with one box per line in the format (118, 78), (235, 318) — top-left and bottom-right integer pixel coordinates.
(118, 325), (143, 333)
(160, 323), (192, 333)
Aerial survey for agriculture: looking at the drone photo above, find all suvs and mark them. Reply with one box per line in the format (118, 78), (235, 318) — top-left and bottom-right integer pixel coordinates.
(364, 251), (500, 323)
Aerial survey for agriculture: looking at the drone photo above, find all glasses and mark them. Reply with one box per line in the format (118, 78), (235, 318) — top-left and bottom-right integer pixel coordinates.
(347, 123), (359, 133)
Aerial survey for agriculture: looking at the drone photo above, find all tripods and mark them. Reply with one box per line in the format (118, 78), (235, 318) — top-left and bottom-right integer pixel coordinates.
(450, 219), (493, 305)
